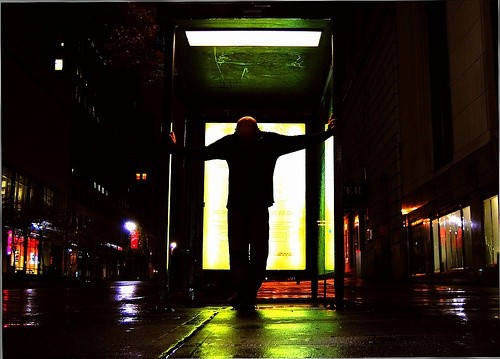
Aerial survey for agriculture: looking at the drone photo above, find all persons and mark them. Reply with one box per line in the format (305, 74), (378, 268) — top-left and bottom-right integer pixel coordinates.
(166, 116), (337, 314)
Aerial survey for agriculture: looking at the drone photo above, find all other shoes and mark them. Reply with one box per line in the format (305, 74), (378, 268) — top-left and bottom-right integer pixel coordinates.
(228, 295), (255, 316)
